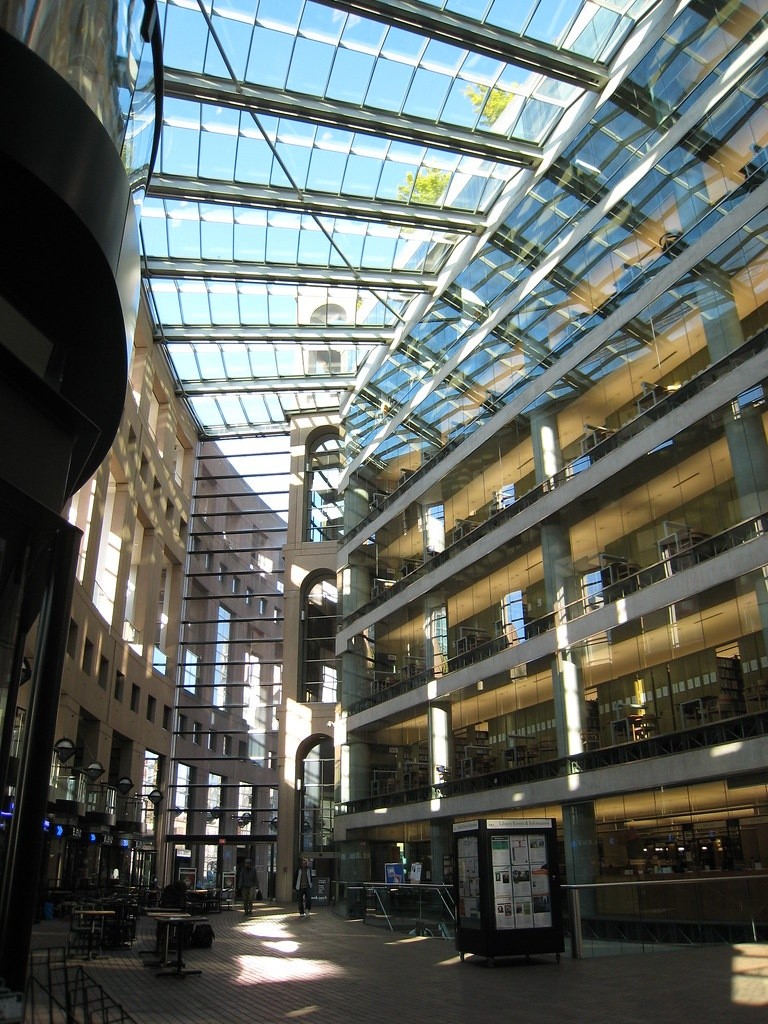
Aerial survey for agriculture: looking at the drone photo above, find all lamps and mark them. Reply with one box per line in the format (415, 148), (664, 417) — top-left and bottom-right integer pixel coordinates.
(263, 817), (278, 828)
(206, 813), (215, 824)
(237, 817), (245, 828)
(125, 790), (164, 804)
(212, 806), (222, 819)
(269, 823), (277, 833)
(59, 762), (106, 781)
(53, 737), (77, 763)
(88, 776), (134, 795)
(232, 813), (252, 825)
(304, 821), (309, 832)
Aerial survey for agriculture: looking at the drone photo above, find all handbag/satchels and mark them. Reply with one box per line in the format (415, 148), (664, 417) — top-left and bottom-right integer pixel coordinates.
(255, 888), (263, 901)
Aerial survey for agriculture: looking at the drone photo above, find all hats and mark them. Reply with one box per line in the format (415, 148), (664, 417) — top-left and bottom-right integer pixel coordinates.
(245, 859), (252, 864)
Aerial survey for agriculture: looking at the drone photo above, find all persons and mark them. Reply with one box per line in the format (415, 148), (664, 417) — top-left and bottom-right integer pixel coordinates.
(292, 860), (312, 919)
(162, 881), (194, 941)
(238, 859), (260, 915)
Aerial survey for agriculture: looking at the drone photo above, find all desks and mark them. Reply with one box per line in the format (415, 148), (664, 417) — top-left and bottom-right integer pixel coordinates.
(502, 735), (539, 769)
(371, 578), (397, 601)
(589, 553), (630, 611)
(658, 519), (697, 579)
(680, 695), (718, 730)
(402, 761), (429, 790)
(579, 423), (608, 456)
(492, 603), (530, 651)
(742, 684), (768, 713)
(460, 746), (496, 780)
(452, 518), (480, 543)
(399, 656), (428, 694)
(610, 703), (647, 746)
(456, 627), (488, 670)
(632, 381), (667, 416)
(371, 670), (401, 705)
(42, 879), (235, 977)
(370, 770), (398, 796)
(369, 140), (768, 600)
(488, 491), (517, 519)
(398, 558), (424, 580)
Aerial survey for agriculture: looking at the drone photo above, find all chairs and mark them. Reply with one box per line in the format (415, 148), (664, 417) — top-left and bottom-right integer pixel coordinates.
(469, 523), (485, 543)
(387, 779), (400, 795)
(468, 632), (492, 663)
(220, 888), (234, 911)
(633, 714), (659, 741)
(383, 677), (401, 699)
(679, 528), (715, 572)
(505, 496), (528, 518)
(67, 902), (105, 957)
(580, 730), (601, 752)
(660, 388), (682, 417)
(207, 888), (223, 913)
(598, 429), (626, 454)
(417, 771), (429, 789)
(538, 739), (558, 761)
(522, 616), (541, 640)
(616, 562), (644, 597)
(411, 664), (427, 687)
(706, 693), (735, 724)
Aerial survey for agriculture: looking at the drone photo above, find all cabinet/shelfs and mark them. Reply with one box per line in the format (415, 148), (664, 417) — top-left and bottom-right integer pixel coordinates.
(398, 632), (768, 876)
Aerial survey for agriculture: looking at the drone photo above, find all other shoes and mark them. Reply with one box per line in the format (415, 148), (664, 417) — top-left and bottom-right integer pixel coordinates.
(304, 909), (310, 917)
(300, 914), (304, 917)
